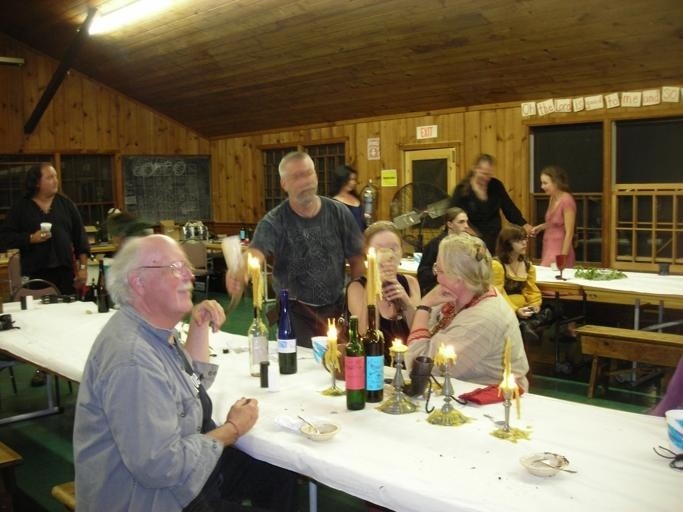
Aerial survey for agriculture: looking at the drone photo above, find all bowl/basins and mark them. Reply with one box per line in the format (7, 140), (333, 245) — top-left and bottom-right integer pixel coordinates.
(521, 451), (570, 478)
(301, 423), (339, 441)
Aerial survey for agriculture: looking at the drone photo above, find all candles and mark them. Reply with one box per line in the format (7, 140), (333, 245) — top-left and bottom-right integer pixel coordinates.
(502, 338), (518, 395)
(249, 254), (267, 312)
(391, 336), (405, 356)
(367, 245), (382, 306)
(326, 315), (339, 373)
(437, 340), (456, 367)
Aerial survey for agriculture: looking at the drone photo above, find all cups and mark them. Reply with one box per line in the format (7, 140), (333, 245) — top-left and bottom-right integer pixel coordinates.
(555, 254), (566, 279)
(311, 336), (326, 362)
(41, 222), (52, 235)
(382, 280), (405, 320)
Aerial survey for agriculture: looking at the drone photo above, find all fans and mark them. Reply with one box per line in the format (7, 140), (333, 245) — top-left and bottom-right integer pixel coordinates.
(389, 181), (451, 253)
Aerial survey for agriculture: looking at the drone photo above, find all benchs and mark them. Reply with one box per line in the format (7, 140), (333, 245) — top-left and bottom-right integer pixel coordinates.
(577, 324), (683, 399)
(49, 479), (80, 511)
(1, 438), (25, 512)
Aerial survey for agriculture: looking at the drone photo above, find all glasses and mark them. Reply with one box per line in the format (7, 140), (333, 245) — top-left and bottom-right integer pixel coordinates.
(138, 262), (186, 277)
(432, 263), (444, 276)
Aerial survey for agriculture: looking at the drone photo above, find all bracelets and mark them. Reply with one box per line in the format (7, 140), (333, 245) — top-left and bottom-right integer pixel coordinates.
(80, 264), (85, 270)
(417, 305), (432, 314)
(226, 418), (240, 444)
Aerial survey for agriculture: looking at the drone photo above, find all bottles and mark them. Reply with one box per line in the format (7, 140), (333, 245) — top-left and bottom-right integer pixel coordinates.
(247, 307), (270, 377)
(344, 315), (367, 411)
(361, 304), (385, 403)
(96, 258), (110, 313)
(277, 289), (298, 375)
(238, 223), (246, 241)
(248, 225), (254, 242)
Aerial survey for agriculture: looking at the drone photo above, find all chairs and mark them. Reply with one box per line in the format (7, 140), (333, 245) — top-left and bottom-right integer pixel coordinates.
(14, 280), (61, 299)
(178, 239), (210, 302)
(8, 251), (21, 303)
(183, 219), (209, 239)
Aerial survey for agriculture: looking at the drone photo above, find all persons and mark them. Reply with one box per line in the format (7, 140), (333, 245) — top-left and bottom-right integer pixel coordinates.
(73, 234), (259, 512)
(534, 166), (578, 344)
(417, 207), (473, 299)
(491, 223), (542, 318)
(327, 165), (365, 232)
(446, 154), (532, 256)
(225, 152), (366, 349)
(3, 163), (91, 387)
(405, 234), (530, 395)
(345, 220), (420, 367)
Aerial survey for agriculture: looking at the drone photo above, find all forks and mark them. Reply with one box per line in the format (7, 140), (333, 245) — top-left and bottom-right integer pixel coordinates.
(533, 458), (577, 474)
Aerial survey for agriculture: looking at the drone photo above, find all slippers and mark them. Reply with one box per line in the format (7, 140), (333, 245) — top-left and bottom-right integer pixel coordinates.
(31, 370), (47, 386)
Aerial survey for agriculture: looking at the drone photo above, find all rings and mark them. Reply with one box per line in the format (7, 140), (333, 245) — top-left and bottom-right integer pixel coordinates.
(41, 231), (47, 238)
